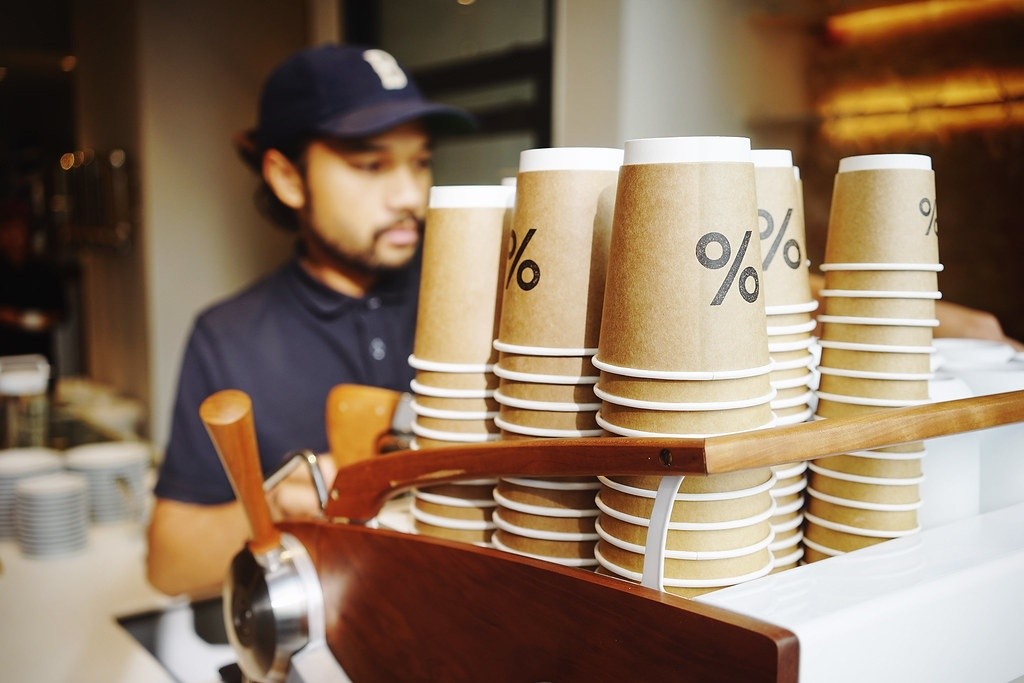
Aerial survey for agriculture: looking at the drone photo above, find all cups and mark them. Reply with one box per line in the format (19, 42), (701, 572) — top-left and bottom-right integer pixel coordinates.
(402, 457), (949, 600)
(404, 132), (937, 452)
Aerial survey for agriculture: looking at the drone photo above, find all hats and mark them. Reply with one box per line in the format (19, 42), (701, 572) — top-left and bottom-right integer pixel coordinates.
(255, 43), (475, 142)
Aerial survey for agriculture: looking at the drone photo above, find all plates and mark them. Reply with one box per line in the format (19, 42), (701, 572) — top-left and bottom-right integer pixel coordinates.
(0, 439), (149, 560)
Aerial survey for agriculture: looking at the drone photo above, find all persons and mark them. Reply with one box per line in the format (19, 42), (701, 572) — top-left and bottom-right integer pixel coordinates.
(148, 41), (475, 600)
